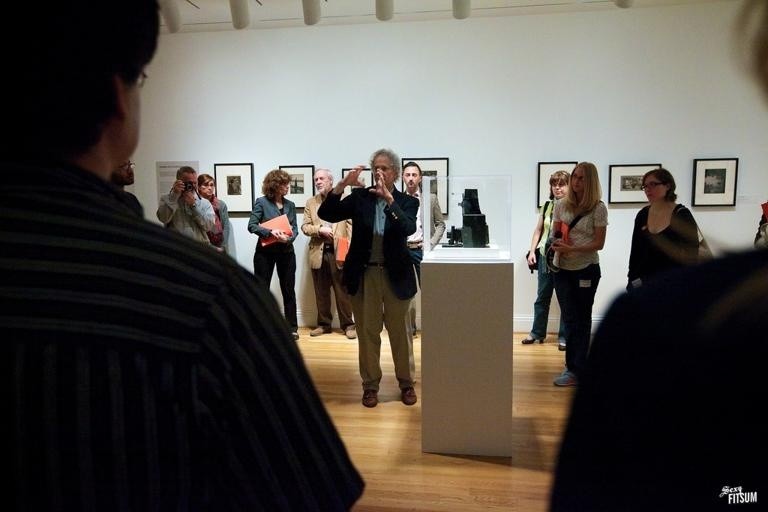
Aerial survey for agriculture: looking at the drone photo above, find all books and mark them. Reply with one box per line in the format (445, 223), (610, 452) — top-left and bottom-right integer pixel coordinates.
(257, 213), (293, 247)
(334, 237), (349, 261)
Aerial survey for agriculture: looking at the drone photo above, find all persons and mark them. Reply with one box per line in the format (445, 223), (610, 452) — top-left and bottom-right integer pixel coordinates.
(521, 170), (567, 352)
(301, 167), (357, 339)
(316, 146), (421, 408)
(198, 173), (228, 255)
(402, 160), (446, 338)
(754, 199), (768, 249)
(247, 169), (299, 339)
(625, 169), (699, 291)
(544, 2), (768, 512)
(113, 157), (142, 218)
(0, 0), (366, 512)
(155, 165), (215, 246)
(554, 162), (609, 386)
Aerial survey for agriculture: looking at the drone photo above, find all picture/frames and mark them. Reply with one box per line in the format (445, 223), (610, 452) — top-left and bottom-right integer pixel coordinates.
(608, 163), (663, 203)
(537, 161), (580, 210)
(400, 156), (450, 217)
(213, 162), (256, 214)
(278, 164), (316, 210)
(692, 158), (738, 206)
(341, 168), (374, 196)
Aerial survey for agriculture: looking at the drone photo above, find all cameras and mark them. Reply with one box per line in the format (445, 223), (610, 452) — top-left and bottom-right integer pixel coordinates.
(183, 181), (193, 191)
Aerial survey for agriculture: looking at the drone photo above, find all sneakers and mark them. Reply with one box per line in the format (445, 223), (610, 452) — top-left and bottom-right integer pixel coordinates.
(553, 370), (577, 387)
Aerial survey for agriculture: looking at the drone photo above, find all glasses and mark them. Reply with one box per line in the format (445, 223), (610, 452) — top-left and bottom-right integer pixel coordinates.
(118, 162), (136, 170)
(640, 181), (664, 191)
(137, 69), (149, 89)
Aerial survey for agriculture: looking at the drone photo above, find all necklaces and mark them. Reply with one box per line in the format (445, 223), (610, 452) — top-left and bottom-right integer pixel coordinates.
(653, 204), (667, 231)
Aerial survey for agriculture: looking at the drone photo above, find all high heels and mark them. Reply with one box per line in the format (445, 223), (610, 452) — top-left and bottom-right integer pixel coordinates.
(559, 342), (567, 352)
(521, 335), (544, 345)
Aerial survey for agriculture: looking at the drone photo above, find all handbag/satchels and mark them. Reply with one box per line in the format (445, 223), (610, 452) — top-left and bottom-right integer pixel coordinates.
(526, 247), (541, 274)
(696, 237), (715, 262)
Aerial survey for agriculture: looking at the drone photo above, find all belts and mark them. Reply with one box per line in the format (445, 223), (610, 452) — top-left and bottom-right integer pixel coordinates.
(407, 241), (422, 249)
(365, 262), (386, 272)
(323, 244), (335, 249)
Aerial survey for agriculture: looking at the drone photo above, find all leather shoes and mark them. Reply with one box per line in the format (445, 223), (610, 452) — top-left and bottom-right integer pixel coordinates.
(400, 386), (417, 406)
(362, 388), (378, 407)
(344, 326), (357, 339)
(290, 332), (299, 340)
(310, 324), (332, 337)
